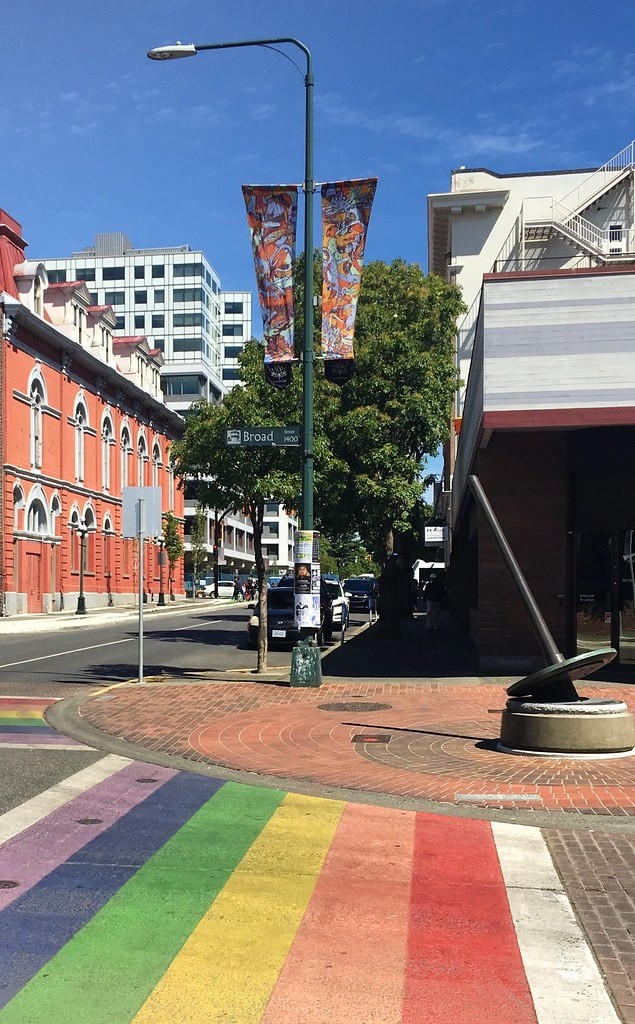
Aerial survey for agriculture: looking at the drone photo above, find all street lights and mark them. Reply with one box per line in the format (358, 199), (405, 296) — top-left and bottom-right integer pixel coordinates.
(147, 35), (324, 688)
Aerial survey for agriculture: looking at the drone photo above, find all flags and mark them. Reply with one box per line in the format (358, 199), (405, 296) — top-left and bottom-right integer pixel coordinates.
(240, 184), (298, 363)
(320, 177), (378, 360)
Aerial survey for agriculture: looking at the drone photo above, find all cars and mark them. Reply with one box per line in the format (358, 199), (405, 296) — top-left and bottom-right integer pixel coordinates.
(247, 586), (332, 650)
(267, 572), (380, 611)
(324, 580), (352, 631)
(341, 578), (379, 614)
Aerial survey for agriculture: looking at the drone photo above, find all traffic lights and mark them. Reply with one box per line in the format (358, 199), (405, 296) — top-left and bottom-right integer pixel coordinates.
(213, 545), (218, 558)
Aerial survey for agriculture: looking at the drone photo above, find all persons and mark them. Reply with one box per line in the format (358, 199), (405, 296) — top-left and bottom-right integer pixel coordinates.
(232, 575), (261, 601)
(422, 574), (445, 630)
(296, 565), (311, 581)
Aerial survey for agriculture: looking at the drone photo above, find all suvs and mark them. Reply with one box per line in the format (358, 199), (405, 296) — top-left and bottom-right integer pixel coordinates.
(196, 580), (236, 599)
